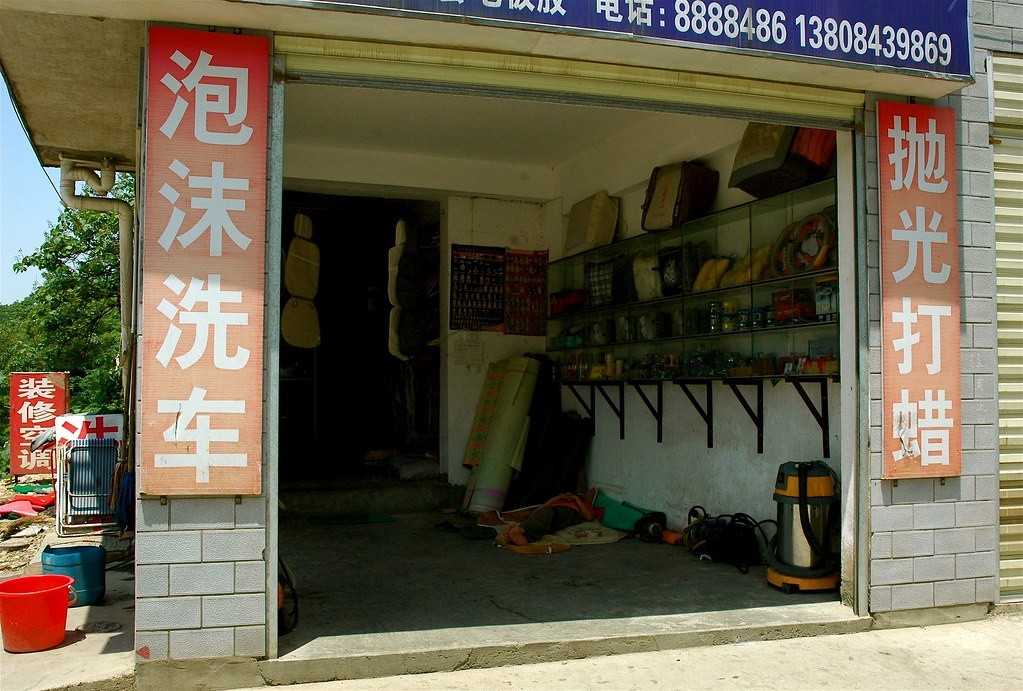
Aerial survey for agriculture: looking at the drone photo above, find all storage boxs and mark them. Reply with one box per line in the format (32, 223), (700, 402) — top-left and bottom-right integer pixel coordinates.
(672, 309), (699, 336)
(802, 360), (838, 375)
(617, 315), (637, 342)
(728, 361), (776, 376)
(771, 287), (813, 326)
(637, 311), (672, 340)
(814, 276), (837, 321)
(589, 319), (616, 344)
(550, 289), (591, 316)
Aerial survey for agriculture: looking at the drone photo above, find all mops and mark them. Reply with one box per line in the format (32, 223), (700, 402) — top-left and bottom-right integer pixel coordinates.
(108, 332), (136, 532)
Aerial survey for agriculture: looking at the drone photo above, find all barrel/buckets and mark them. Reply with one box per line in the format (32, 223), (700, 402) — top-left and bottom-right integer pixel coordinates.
(0, 574), (78, 653)
(41, 545), (107, 607)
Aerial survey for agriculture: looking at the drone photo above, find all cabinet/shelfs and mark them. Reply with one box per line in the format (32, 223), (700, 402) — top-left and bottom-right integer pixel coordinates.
(540, 175), (839, 461)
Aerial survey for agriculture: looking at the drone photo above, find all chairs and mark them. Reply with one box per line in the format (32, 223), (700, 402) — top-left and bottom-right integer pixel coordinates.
(54, 437), (122, 537)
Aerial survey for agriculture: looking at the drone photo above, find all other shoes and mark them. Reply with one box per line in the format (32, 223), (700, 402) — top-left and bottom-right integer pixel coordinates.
(460, 525), (497, 540)
(434, 520), (461, 532)
